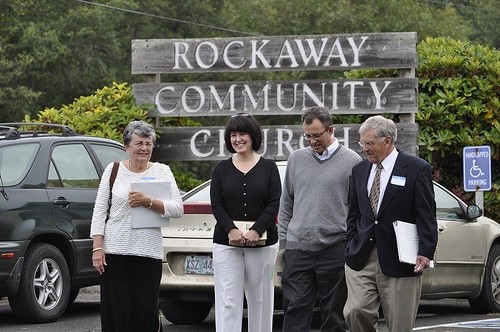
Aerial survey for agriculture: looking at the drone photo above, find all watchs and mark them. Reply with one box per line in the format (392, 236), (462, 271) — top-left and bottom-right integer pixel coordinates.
(147, 198), (154, 210)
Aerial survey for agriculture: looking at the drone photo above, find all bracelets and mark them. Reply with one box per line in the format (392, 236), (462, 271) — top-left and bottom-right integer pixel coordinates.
(92, 247), (103, 252)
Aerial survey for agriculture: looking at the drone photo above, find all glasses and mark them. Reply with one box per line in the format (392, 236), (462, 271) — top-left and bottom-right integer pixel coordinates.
(127, 142), (155, 147)
(231, 113), (252, 118)
(358, 136), (383, 147)
(303, 124), (332, 140)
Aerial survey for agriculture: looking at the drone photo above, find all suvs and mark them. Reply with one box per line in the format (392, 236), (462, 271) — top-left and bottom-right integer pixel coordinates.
(0, 123), (189, 325)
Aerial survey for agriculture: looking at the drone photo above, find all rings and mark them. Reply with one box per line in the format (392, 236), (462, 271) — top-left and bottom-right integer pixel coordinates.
(92, 264), (96, 266)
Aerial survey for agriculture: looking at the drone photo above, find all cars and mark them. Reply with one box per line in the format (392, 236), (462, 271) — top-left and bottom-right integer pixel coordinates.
(158, 158), (500, 324)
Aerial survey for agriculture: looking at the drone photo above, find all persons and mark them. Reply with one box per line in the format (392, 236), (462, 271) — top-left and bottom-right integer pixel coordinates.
(210, 113), (282, 332)
(277, 106), (363, 332)
(90, 120), (185, 332)
(343, 115), (438, 332)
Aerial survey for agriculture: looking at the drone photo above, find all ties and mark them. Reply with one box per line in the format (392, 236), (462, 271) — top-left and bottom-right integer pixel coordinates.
(369, 164), (383, 219)
